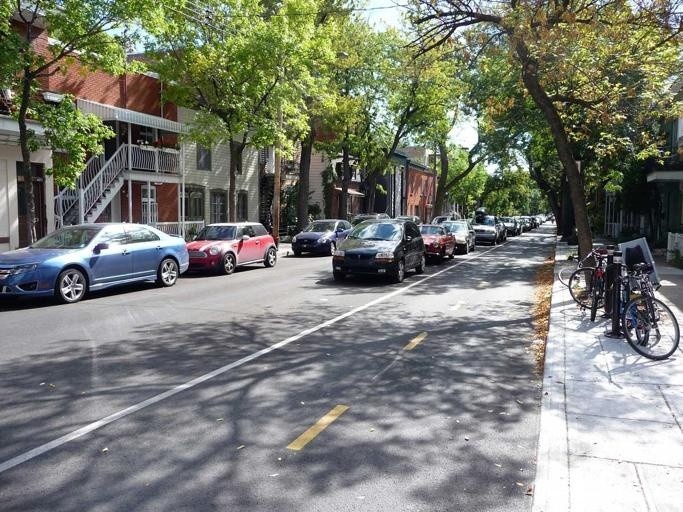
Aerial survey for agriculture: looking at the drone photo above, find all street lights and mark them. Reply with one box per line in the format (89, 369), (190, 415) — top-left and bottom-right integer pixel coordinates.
(432, 147), (469, 214)
(273, 52), (348, 247)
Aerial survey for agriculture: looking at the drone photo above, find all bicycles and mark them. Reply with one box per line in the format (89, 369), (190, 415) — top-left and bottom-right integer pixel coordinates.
(558, 245), (679, 360)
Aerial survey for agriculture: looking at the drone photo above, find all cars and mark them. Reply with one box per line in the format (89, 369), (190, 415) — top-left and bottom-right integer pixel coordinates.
(291, 206), (555, 283)
(0, 222), (278, 303)
